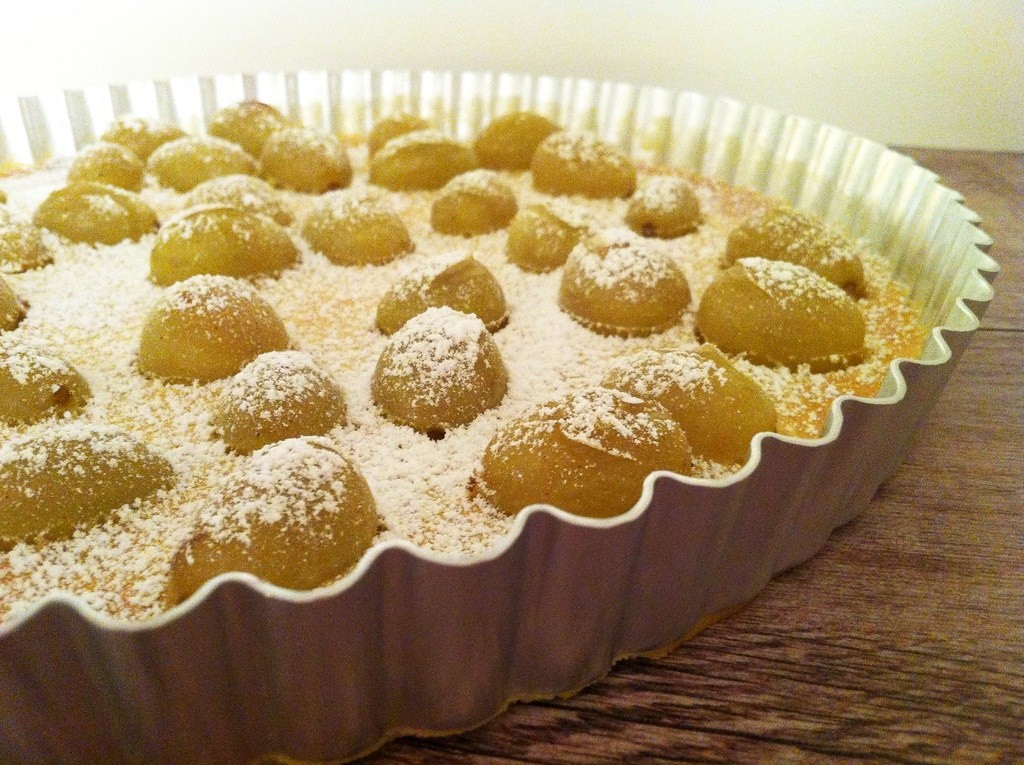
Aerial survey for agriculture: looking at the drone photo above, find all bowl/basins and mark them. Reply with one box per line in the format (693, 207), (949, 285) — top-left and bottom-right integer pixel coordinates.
(0, 67), (998, 765)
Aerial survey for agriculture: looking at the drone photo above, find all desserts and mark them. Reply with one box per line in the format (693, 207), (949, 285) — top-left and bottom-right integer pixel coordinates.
(1, 69), (999, 764)
